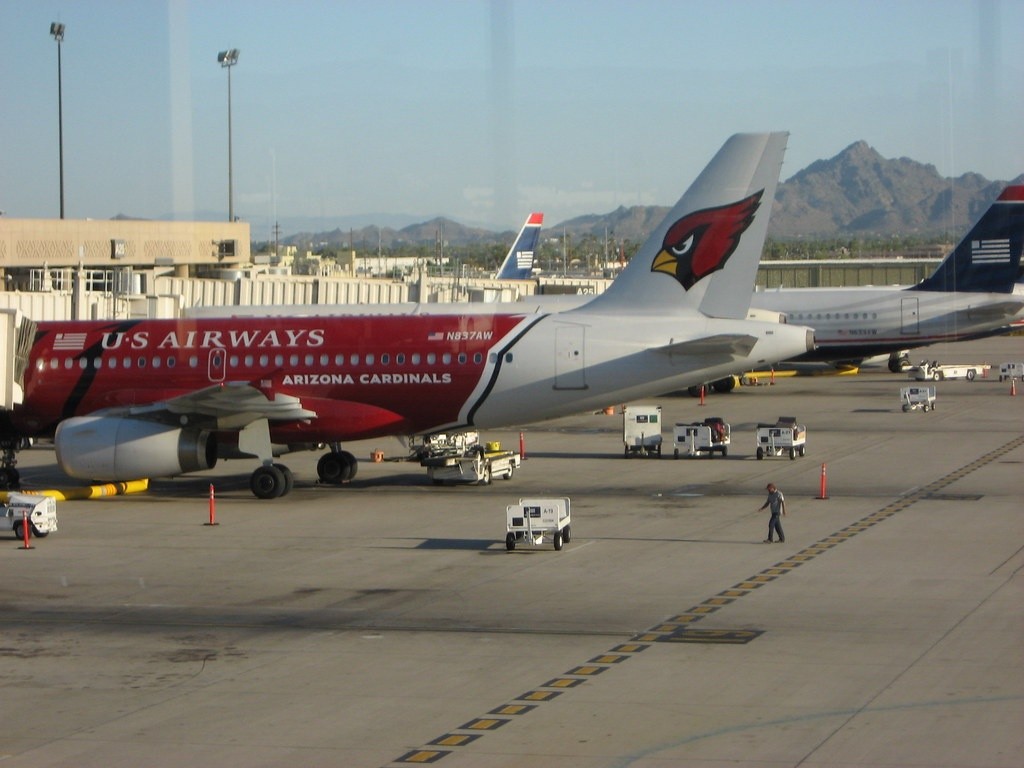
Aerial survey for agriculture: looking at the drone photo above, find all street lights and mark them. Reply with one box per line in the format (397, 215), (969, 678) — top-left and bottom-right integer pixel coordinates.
(49, 22), (64, 223)
(218, 49), (242, 224)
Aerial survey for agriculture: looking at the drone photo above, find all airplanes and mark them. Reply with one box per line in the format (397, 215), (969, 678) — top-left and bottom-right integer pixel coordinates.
(1, 128), (1024, 502)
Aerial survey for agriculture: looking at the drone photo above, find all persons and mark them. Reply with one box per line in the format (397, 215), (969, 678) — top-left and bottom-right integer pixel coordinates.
(758, 483), (787, 544)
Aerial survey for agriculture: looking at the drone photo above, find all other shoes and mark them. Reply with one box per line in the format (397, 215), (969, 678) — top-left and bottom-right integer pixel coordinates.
(775, 541), (784, 543)
(763, 539), (772, 544)
